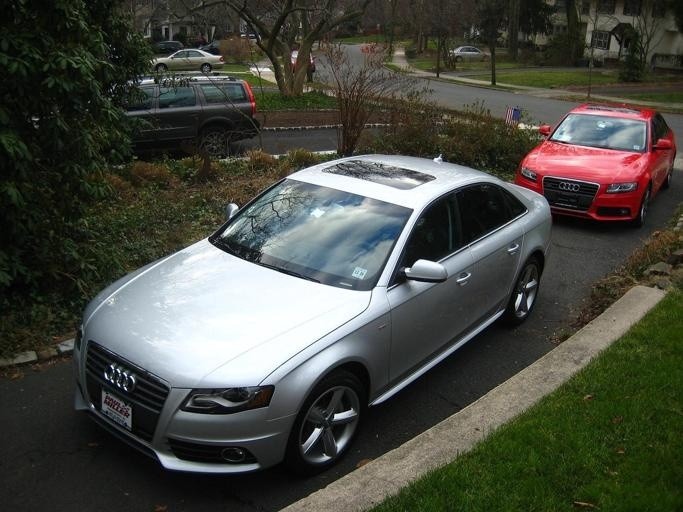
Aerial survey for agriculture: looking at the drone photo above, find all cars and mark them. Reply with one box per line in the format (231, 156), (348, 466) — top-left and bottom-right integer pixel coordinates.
(515, 103), (676, 225)
(148, 49), (224, 73)
(290, 49), (316, 73)
(448, 46), (490, 62)
(72, 155), (552, 475)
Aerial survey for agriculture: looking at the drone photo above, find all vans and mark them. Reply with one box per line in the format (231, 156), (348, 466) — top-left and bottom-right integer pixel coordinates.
(108, 76), (260, 155)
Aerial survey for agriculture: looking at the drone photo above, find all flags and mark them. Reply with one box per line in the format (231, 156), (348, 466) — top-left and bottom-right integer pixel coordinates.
(505, 109), (522, 129)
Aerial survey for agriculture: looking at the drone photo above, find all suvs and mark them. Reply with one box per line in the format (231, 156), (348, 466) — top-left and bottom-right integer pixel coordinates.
(230, 34), (261, 44)
(155, 41), (184, 54)
